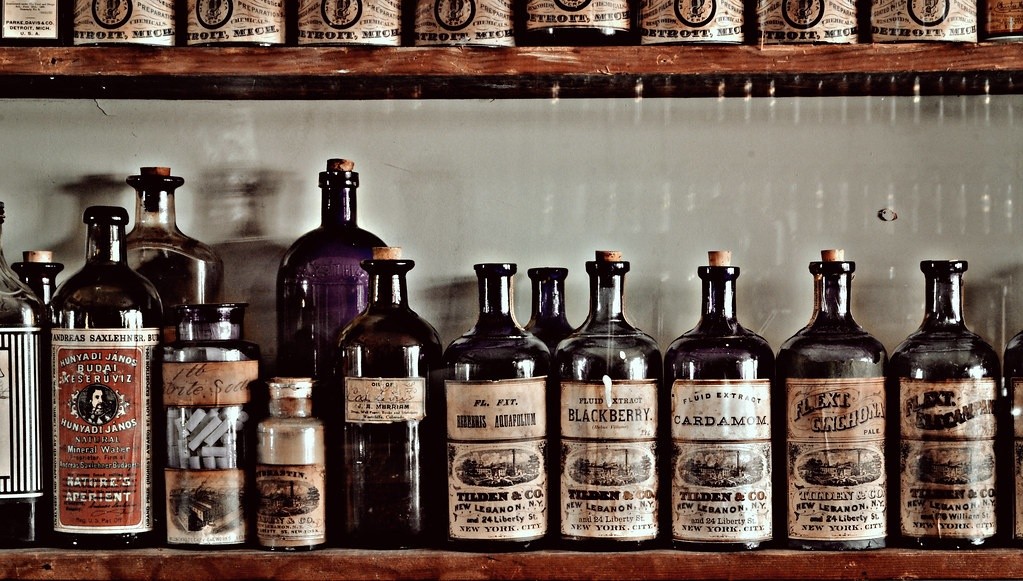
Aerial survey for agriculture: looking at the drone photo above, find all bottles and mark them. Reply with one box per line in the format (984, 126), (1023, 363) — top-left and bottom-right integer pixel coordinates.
(523, 267), (575, 356)
(442, 263), (551, 552)
(891, 259), (1000, 551)
(256, 377), (327, 551)
(275, 159), (388, 548)
(44, 206), (163, 551)
(1001, 330), (1022, 550)
(662, 251), (775, 553)
(125, 166), (225, 308)
(775, 249), (891, 552)
(336, 247), (442, 550)
(552, 250), (661, 552)
(10, 250), (65, 308)
(0, 202), (49, 549)
(0, 0), (1023, 46)
(149, 304), (262, 549)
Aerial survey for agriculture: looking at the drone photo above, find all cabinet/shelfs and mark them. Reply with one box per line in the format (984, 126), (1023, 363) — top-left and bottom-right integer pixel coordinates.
(0, 0), (1023, 581)
